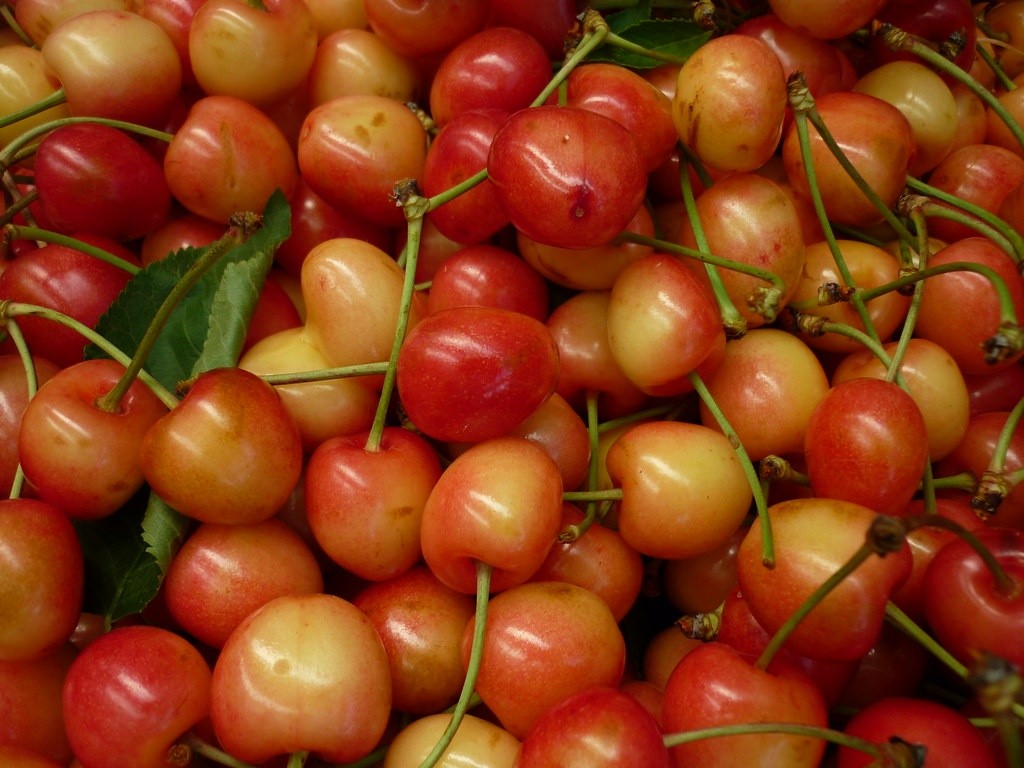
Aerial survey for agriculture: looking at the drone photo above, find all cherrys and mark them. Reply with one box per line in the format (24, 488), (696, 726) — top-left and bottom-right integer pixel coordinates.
(0, 0), (1024, 768)
